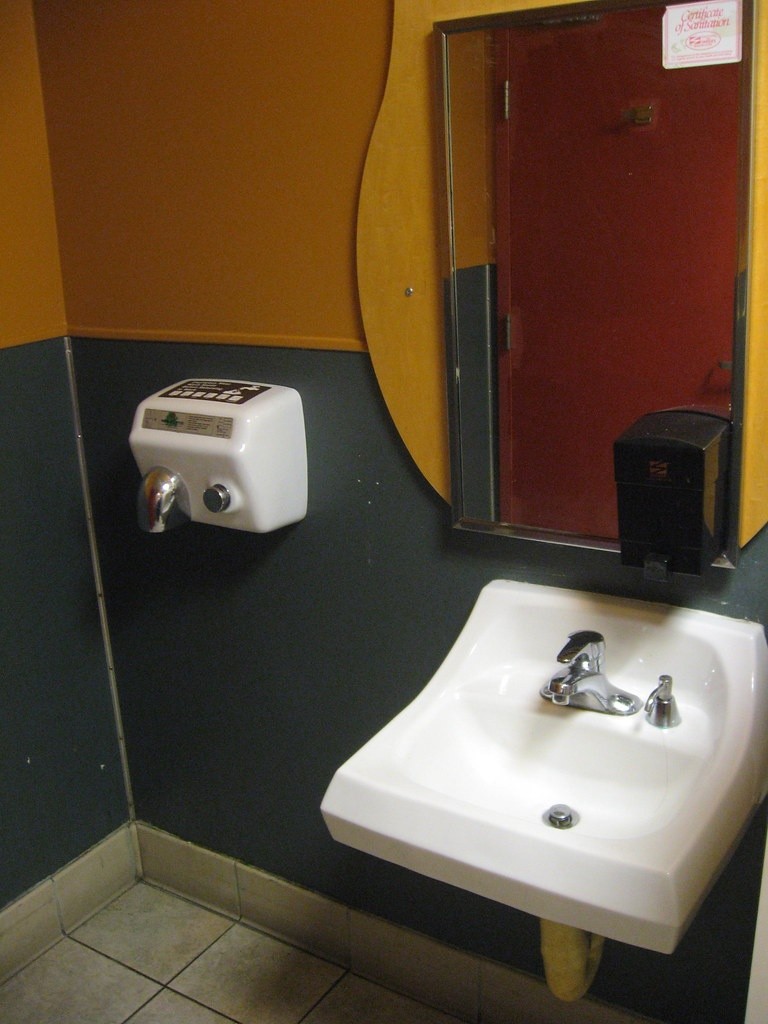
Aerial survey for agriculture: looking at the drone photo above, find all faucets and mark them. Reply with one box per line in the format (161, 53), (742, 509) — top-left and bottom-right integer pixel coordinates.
(539, 629), (646, 717)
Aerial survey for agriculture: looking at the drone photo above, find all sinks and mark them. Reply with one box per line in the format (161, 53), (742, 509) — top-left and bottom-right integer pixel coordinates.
(319, 678), (760, 957)
